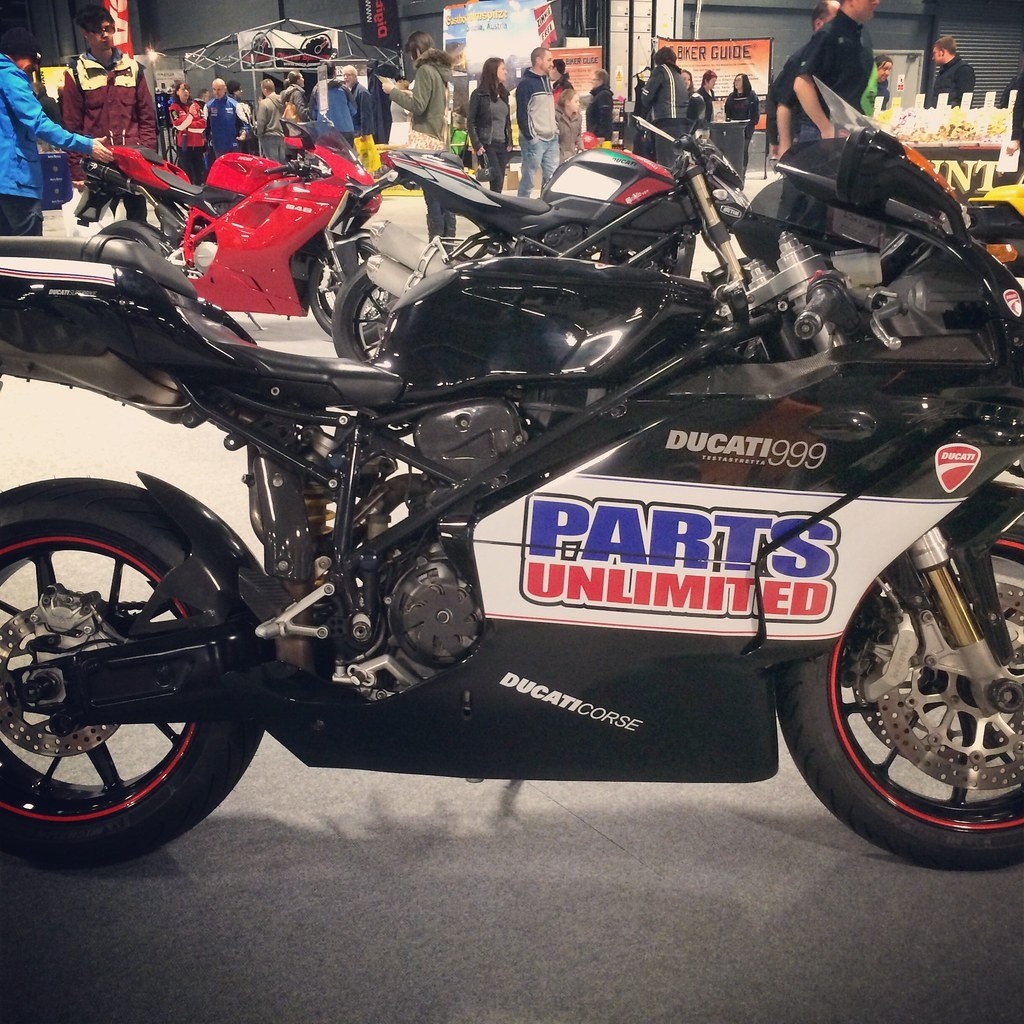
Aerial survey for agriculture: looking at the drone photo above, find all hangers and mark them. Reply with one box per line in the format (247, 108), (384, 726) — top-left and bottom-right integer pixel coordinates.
(632, 60), (654, 77)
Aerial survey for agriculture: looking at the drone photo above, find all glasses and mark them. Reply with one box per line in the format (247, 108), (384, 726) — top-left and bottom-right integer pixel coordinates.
(89, 24), (116, 35)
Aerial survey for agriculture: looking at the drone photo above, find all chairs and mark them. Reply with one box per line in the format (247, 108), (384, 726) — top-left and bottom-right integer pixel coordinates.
(449, 129), (468, 162)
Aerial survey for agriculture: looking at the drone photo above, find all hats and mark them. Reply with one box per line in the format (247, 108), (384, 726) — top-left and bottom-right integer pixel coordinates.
(0, 27), (42, 64)
(226, 80), (241, 95)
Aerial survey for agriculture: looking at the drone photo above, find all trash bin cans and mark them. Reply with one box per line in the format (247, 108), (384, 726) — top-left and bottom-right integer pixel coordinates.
(705, 121), (748, 184)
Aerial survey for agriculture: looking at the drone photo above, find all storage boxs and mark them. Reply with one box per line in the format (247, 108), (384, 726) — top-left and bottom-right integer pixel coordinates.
(507, 163), (543, 190)
(563, 37), (590, 48)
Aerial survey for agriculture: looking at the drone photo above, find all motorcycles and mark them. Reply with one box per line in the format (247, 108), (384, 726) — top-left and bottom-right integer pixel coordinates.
(68, 96), (407, 344)
(0, 72), (1024, 873)
(369, 92), (751, 303)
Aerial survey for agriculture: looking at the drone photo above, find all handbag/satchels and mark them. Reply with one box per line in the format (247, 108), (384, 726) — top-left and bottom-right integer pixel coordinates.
(354, 133), (381, 172)
(404, 130), (449, 155)
(476, 152), (494, 181)
(592, 140), (612, 151)
(279, 103), (303, 124)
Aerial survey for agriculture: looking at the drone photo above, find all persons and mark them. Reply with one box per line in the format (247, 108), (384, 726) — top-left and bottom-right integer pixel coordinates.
(992, 53), (1024, 187)
(771, 0), (880, 161)
(0, 26), (114, 236)
(31, 82), (65, 127)
(585, 69), (613, 141)
(555, 88), (583, 163)
(515, 47), (559, 199)
(873, 55), (893, 111)
(681, 69), (694, 96)
(308, 61), (358, 150)
(383, 30), (455, 252)
(279, 70), (311, 163)
(257, 78), (286, 164)
(548, 58), (574, 103)
(342, 65), (375, 139)
(697, 69), (718, 122)
(641, 46), (691, 168)
(466, 57), (513, 195)
(62, 4), (158, 223)
(927, 37), (975, 110)
(725, 73), (760, 183)
(168, 77), (253, 186)
(387, 79), (414, 146)
